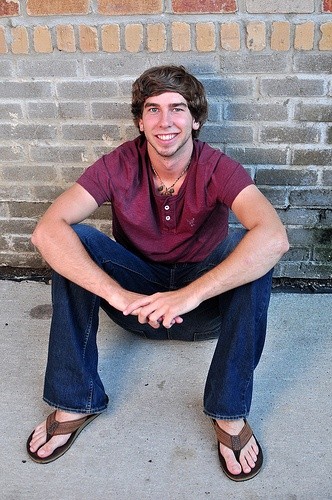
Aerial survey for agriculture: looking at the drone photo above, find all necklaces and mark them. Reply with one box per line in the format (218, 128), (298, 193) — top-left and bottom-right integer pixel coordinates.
(148, 160), (190, 197)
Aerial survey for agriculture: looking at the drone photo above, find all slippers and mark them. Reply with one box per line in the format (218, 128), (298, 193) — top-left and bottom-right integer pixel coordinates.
(26, 397), (102, 464)
(210, 417), (263, 482)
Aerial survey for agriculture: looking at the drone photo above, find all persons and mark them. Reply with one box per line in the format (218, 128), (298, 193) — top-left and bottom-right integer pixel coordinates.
(26, 65), (289, 482)
